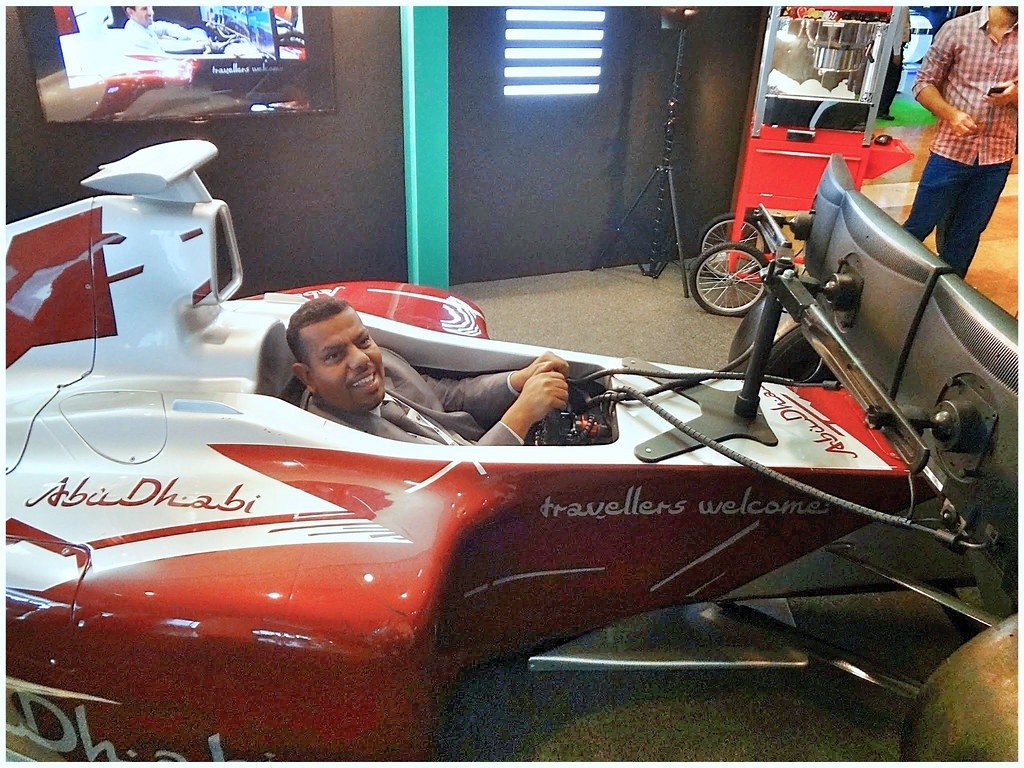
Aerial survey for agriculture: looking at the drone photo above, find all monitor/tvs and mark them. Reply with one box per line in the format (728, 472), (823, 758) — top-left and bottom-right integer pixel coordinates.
(793, 152), (1018, 579)
(15, 6), (331, 127)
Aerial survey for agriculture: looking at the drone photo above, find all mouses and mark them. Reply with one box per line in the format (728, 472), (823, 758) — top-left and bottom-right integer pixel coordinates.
(875, 135), (892, 145)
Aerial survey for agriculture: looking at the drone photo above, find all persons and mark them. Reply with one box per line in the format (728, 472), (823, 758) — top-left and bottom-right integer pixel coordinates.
(286, 297), (570, 446)
(121, 6), (210, 56)
(902, 6), (1018, 281)
(876, 6), (911, 120)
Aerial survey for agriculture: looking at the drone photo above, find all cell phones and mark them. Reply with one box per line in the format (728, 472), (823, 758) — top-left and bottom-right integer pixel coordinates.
(986, 85), (1006, 96)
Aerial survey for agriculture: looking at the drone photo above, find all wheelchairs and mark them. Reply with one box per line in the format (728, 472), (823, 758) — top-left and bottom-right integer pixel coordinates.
(686, 202), (809, 317)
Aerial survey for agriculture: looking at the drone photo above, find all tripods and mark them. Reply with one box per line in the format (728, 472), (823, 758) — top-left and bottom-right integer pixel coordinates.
(589, 16), (692, 300)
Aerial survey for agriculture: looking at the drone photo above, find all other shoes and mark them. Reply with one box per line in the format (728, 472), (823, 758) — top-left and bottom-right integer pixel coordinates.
(878, 110), (895, 120)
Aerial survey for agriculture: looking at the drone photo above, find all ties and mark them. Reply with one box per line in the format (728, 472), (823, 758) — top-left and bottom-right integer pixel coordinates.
(379, 401), (448, 445)
